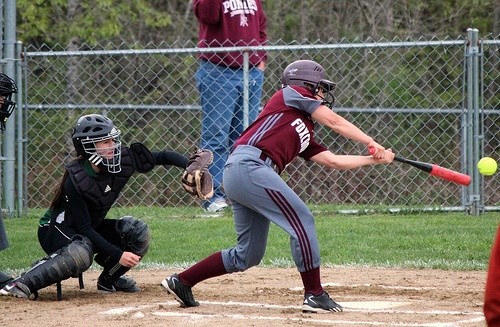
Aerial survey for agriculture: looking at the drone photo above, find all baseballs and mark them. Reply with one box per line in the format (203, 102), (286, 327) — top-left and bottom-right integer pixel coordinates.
(477, 157), (498, 176)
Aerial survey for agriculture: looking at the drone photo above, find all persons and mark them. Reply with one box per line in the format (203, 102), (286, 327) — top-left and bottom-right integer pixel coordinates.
(159, 59), (395, 313)
(0, 72), (19, 291)
(0, 114), (215, 300)
(194, 0), (267, 215)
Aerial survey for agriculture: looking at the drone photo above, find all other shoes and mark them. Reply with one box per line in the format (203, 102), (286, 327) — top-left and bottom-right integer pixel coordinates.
(208, 200), (228, 213)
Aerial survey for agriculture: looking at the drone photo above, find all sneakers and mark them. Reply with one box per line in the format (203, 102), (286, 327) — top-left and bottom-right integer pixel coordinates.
(97, 275), (140, 292)
(0, 283), (28, 300)
(161, 273), (196, 308)
(302, 291), (343, 314)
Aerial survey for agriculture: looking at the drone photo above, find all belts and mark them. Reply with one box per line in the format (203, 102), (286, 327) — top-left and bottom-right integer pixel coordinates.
(259, 151), (279, 174)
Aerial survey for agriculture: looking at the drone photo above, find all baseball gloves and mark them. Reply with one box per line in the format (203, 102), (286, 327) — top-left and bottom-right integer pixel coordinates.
(182, 148), (214, 200)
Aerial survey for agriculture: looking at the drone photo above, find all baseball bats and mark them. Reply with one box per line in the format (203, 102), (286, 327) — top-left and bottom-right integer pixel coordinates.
(368, 146), (471, 186)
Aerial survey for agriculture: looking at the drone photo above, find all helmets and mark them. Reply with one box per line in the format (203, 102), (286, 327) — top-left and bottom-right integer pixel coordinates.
(70, 114), (122, 173)
(0, 72), (19, 131)
(281, 59), (336, 92)
